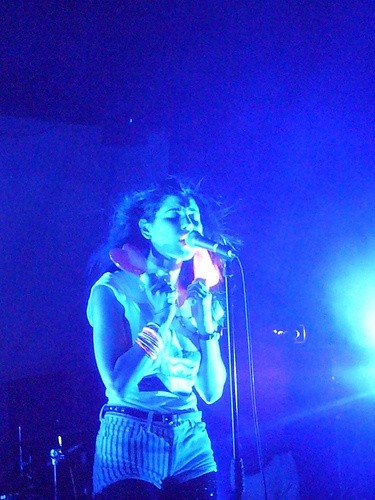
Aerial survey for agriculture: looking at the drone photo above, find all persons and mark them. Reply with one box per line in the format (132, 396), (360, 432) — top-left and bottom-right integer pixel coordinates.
(85, 174), (227, 500)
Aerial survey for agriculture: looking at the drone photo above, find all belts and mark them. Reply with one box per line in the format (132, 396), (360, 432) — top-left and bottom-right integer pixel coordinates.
(103, 405), (202, 422)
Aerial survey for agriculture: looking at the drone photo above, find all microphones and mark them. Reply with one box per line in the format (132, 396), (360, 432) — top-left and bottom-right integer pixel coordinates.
(186, 229), (237, 260)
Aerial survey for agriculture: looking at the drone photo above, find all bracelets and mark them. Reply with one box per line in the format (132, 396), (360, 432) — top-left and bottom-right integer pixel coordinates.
(196, 330), (222, 341)
(135, 322), (165, 361)
